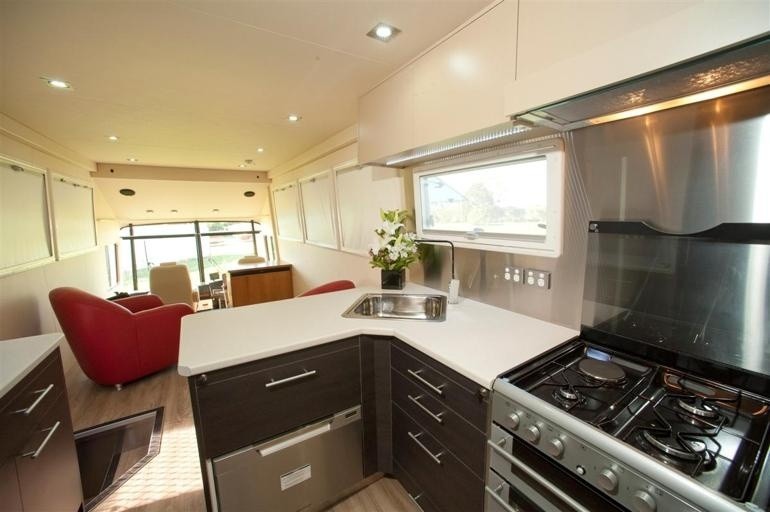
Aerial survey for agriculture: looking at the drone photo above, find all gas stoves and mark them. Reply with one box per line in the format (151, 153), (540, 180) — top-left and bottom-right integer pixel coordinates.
(497, 333), (770, 511)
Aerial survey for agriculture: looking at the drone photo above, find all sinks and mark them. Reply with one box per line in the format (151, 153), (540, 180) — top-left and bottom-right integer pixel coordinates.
(340, 292), (448, 323)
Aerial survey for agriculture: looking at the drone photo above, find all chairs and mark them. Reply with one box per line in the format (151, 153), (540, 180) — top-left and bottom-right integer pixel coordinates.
(239, 256), (265, 263)
(49, 286), (194, 391)
(298, 280), (355, 297)
(149, 262), (200, 306)
(209, 273), (225, 308)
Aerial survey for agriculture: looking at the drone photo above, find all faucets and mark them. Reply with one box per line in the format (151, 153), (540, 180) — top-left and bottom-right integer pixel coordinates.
(413, 238), (461, 305)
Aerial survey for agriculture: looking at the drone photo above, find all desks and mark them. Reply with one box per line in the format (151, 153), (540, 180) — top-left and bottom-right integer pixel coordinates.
(212, 289), (225, 308)
(227, 261), (293, 308)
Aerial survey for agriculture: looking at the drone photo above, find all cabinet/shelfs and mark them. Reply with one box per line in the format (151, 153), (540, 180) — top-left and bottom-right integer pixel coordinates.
(0, 347), (84, 512)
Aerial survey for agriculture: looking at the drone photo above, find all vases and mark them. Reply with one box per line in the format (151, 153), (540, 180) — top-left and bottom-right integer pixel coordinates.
(381, 269), (406, 290)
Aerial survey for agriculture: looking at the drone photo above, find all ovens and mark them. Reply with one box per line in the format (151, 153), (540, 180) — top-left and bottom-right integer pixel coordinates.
(483, 389), (709, 511)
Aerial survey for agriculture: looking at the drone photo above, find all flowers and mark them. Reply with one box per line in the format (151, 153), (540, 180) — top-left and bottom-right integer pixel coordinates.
(368, 207), (422, 271)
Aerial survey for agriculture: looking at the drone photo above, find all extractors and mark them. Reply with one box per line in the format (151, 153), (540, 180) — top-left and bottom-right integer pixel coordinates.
(511, 37), (770, 134)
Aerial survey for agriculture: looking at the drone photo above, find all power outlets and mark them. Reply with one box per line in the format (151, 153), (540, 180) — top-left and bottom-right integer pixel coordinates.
(503, 265), (524, 284)
(526, 268), (551, 289)
